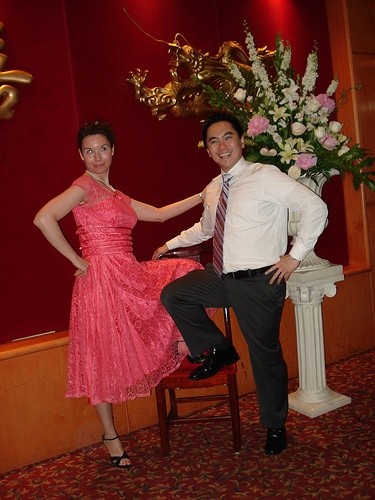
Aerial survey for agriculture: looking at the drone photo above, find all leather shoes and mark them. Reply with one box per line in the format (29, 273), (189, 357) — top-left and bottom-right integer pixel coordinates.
(265, 425), (287, 455)
(188, 338), (241, 380)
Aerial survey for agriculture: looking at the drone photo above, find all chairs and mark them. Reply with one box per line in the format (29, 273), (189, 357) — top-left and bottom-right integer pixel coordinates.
(155, 246), (241, 453)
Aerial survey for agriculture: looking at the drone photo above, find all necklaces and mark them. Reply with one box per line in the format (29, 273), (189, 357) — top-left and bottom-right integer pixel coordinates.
(85, 170), (115, 193)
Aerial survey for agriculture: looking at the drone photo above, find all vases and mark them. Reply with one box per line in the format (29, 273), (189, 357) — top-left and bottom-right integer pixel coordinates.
(287, 168), (340, 273)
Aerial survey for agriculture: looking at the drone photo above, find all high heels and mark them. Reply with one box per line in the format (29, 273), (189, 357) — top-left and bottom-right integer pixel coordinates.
(102, 434), (132, 471)
(186, 354), (207, 364)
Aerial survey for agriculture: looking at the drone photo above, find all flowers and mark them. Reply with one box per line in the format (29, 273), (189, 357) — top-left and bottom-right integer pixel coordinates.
(191, 18), (375, 193)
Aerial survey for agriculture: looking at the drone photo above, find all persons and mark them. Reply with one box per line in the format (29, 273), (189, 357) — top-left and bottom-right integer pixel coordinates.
(33, 119), (214, 468)
(153, 112), (329, 455)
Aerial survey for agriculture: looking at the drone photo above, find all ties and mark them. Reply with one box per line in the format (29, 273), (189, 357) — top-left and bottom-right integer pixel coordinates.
(212, 174), (234, 279)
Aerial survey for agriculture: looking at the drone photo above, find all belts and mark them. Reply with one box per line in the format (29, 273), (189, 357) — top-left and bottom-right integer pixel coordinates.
(222, 264), (274, 280)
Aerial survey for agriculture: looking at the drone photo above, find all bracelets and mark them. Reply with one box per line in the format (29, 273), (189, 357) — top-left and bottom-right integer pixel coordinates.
(198, 192), (205, 203)
